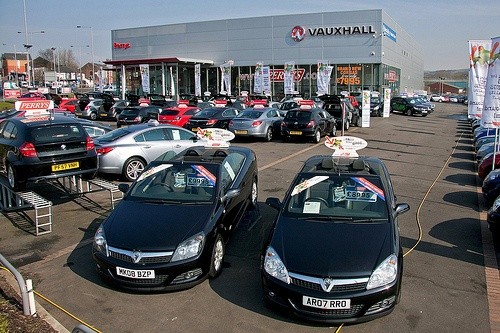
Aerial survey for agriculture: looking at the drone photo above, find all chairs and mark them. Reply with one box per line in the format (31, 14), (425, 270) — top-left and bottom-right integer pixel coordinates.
(164, 168), (187, 193)
(304, 182), (335, 208)
(197, 171), (215, 195)
(346, 181), (380, 211)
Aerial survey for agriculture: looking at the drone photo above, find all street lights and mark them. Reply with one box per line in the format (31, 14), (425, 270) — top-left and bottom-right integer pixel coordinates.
(17, 30), (45, 90)
(76, 25), (95, 89)
(70, 45), (90, 92)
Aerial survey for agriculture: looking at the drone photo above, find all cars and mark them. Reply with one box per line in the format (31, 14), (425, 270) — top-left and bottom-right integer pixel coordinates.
(259, 153), (410, 327)
(389, 95), (436, 117)
(90, 145), (259, 295)
(20, 81), (38, 90)
(95, 83), (129, 94)
(90, 119), (230, 183)
(17, 91), (383, 143)
(469, 117), (500, 231)
(430, 92), (468, 105)
(0, 107), (116, 192)
